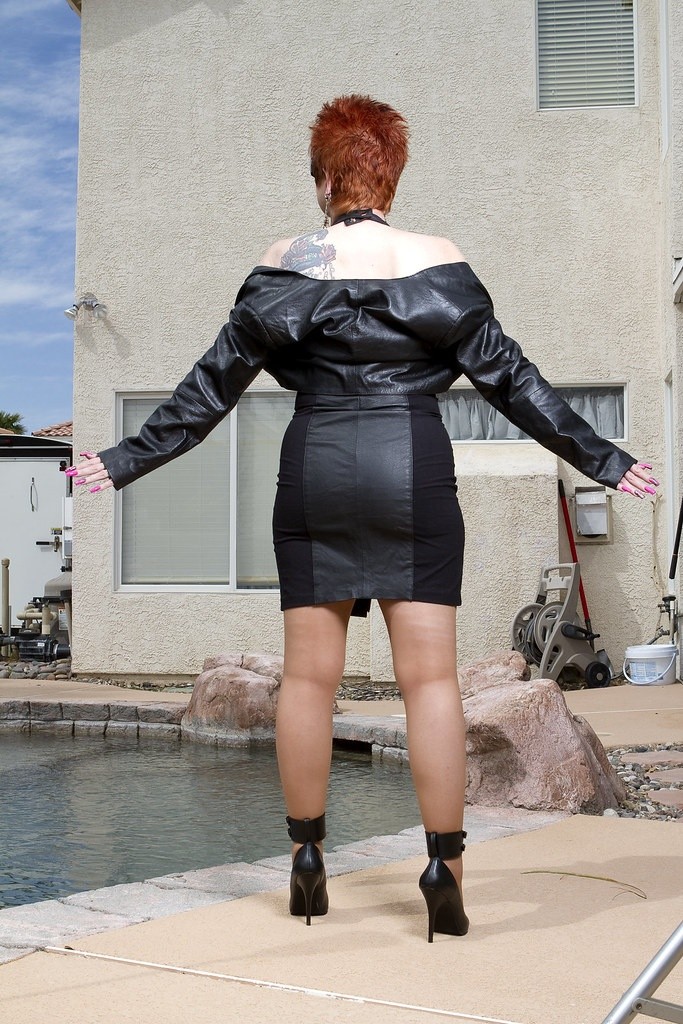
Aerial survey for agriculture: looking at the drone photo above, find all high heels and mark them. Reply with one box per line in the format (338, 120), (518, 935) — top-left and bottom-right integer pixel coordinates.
(285, 812), (329, 926)
(418, 830), (470, 944)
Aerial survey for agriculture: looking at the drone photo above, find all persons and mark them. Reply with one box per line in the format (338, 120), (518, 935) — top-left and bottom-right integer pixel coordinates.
(65, 93), (658, 943)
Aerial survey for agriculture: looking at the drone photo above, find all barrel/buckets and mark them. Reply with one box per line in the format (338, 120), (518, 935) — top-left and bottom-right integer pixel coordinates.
(623, 643), (679, 685)
